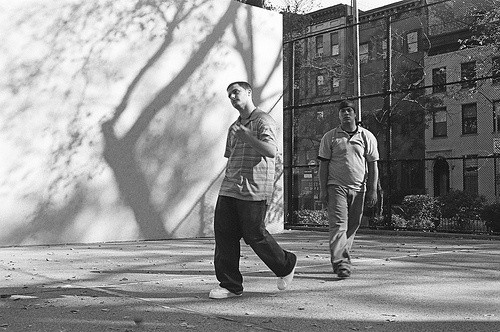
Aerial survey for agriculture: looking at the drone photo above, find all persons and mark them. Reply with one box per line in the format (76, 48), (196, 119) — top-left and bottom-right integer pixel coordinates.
(316, 101), (380, 277)
(209, 81), (297, 298)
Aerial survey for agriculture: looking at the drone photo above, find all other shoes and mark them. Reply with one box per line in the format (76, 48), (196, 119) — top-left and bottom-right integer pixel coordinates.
(337, 268), (351, 277)
(276, 253), (297, 290)
(209, 285), (243, 299)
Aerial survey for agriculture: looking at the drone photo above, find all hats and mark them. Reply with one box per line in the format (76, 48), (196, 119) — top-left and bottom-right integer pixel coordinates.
(338, 100), (356, 113)
